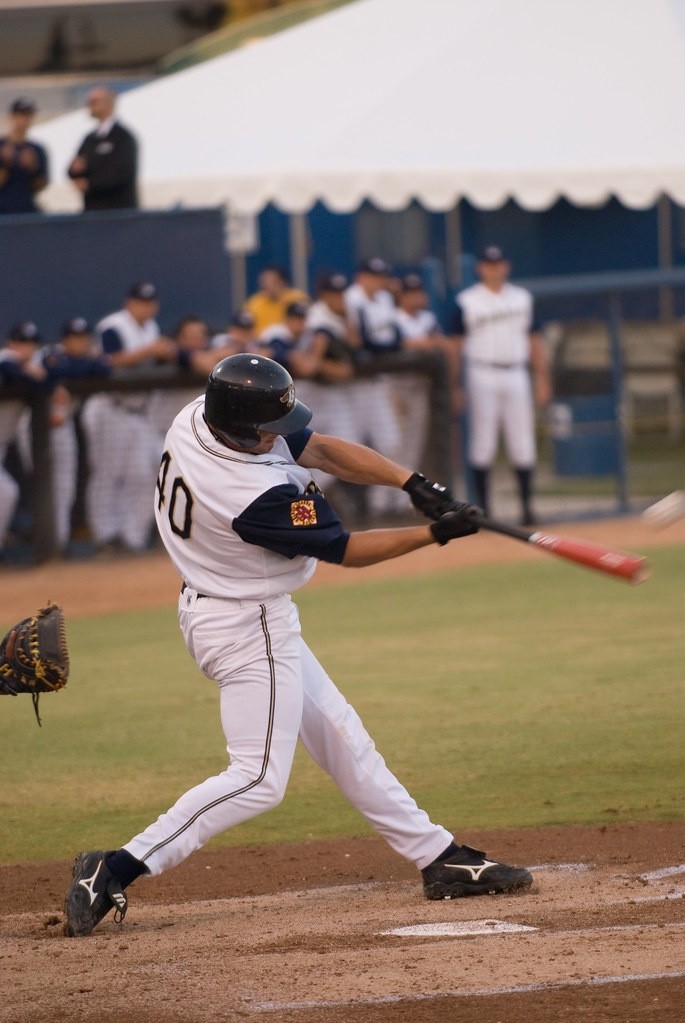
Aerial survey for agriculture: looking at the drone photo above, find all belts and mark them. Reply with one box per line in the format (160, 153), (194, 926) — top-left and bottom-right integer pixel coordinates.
(180, 581), (206, 598)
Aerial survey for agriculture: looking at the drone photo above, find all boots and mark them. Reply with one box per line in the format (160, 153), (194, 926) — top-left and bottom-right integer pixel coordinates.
(472, 468), (488, 518)
(515, 470), (534, 526)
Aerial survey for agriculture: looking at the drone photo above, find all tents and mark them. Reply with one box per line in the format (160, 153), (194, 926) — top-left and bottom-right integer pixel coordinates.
(0, 0), (684, 439)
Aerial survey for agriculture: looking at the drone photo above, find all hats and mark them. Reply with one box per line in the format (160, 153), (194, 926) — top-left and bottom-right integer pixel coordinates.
(480, 245), (503, 263)
(360, 256), (387, 275)
(129, 280), (159, 300)
(319, 272), (352, 292)
(62, 317), (93, 337)
(9, 322), (41, 341)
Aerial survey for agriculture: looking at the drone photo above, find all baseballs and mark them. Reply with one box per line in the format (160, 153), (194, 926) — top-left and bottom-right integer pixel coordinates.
(642, 490), (685, 530)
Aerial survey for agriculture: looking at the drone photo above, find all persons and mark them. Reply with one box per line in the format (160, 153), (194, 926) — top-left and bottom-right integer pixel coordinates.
(3, 247), (552, 572)
(69, 87), (139, 211)
(59, 351), (540, 934)
(0, 95), (47, 216)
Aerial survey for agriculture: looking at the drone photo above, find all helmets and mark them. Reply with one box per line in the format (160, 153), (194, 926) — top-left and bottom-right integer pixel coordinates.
(204, 353), (312, 449)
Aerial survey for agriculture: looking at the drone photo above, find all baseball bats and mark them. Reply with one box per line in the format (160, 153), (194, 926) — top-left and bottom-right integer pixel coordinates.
(466, 513), (652, 587)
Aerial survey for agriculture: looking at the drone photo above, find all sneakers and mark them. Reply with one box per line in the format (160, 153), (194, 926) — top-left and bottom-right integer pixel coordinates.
(421, 844), (533, 900)
(63, 849), (128, 937)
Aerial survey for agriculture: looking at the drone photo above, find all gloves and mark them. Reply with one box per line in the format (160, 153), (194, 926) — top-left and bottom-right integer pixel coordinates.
(430, 503), (484, 546)
(402, 472), (453, 521)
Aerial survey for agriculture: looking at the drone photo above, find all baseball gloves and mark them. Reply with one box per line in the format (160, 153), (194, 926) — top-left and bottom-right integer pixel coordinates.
(0, 598), (70, 728)
